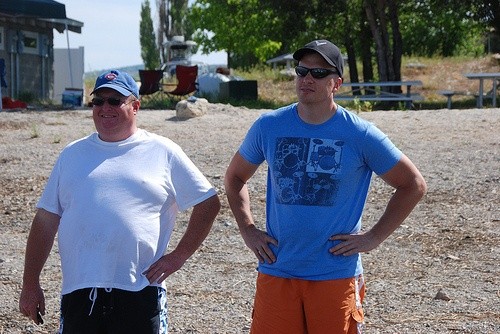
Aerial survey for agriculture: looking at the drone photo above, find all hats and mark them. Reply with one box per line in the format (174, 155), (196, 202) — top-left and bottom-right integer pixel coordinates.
(90, 70), (139, 98)
(293, 40), (343, 76)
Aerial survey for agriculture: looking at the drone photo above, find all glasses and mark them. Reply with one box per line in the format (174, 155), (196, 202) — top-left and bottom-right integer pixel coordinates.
(295, 66), (338, 79)
(92, 98), (135, 106)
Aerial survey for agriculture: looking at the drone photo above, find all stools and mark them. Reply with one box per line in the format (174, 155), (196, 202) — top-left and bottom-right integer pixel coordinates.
(468, 92), (488, 109)
(436, 89), (466, 110)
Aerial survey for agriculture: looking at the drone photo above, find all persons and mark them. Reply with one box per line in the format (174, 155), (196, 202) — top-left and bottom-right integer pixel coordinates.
(223, 39), (428, 334)
(19, 70), (221, 334)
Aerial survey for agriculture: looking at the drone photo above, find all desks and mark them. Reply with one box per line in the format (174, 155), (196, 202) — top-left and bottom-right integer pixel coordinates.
(465, 73), (500, 109)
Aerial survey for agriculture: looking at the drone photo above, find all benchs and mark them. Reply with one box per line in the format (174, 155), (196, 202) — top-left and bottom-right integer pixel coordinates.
(333, 80), (425, 111)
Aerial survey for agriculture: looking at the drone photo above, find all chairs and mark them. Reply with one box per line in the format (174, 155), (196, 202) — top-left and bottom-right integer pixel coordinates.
(167, 65), (197, 110)
(136, 70), (163, 110)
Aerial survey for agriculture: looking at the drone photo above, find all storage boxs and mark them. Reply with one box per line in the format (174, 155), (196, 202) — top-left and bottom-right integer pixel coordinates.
(63, 87), (83, 106)
